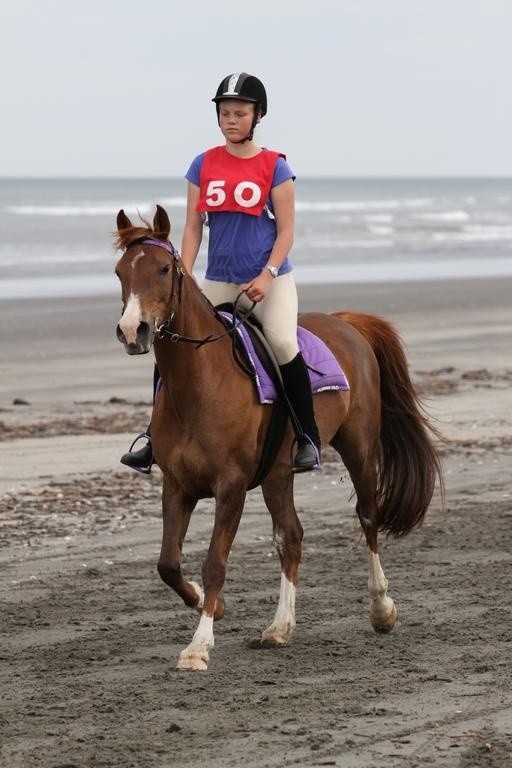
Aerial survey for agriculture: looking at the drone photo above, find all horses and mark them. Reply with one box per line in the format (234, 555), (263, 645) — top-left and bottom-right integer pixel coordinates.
(109, 204), (452, 673)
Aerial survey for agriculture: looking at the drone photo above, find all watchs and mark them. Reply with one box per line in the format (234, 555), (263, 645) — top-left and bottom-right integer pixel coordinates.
(262, 264), (280, 278)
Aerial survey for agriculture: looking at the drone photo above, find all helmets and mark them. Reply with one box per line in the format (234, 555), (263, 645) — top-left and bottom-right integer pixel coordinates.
(212, 72), (267, 117)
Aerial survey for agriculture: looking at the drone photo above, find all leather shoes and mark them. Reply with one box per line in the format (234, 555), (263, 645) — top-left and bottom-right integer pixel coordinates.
(294, 443), (322, 468)
(121, 442), (156, 467)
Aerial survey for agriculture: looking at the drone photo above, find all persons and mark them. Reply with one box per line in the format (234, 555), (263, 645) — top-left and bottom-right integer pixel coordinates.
(120, 71), (324, 474)
